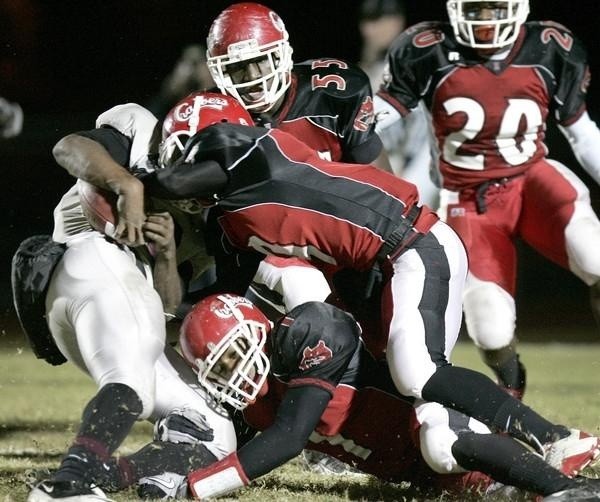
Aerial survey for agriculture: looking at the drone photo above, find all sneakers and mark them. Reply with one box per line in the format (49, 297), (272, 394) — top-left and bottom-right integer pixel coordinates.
(539, 474), (599, 502)
(27, 479), (117, 502)
(536, 427), (600, 500)
(92, 455), (133, 493)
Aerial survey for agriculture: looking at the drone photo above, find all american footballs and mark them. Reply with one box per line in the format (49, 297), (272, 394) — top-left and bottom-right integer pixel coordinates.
(79, 177), (141, 248)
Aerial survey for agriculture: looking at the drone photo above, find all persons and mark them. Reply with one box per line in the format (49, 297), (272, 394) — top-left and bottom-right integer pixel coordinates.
(370, 1), (598, 400)
(1, 2), (599, 500)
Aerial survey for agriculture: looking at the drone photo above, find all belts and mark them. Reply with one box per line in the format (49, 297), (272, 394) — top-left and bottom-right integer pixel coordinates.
(375, 204), (422, 265)
(474, 172), (525, 214)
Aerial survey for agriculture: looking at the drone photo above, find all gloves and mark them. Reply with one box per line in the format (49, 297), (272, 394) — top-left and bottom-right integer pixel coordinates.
(154, 407), (214, 445)
(135, 471), (189, 502)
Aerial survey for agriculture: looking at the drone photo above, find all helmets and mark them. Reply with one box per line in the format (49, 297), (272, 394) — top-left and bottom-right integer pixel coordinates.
(158, 93), (256, 215)
(206, 2), (294, 115)
(446, 0), (531, 50)
(178, 293), (275, 411)
